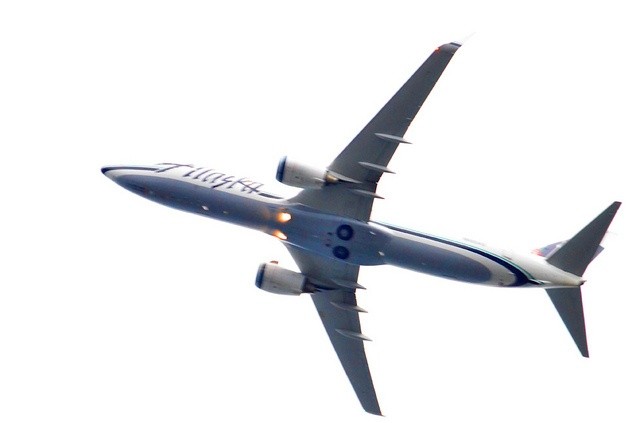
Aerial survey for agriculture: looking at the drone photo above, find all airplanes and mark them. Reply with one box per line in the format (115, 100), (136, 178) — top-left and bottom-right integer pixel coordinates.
(100, 40), (622, 417)
